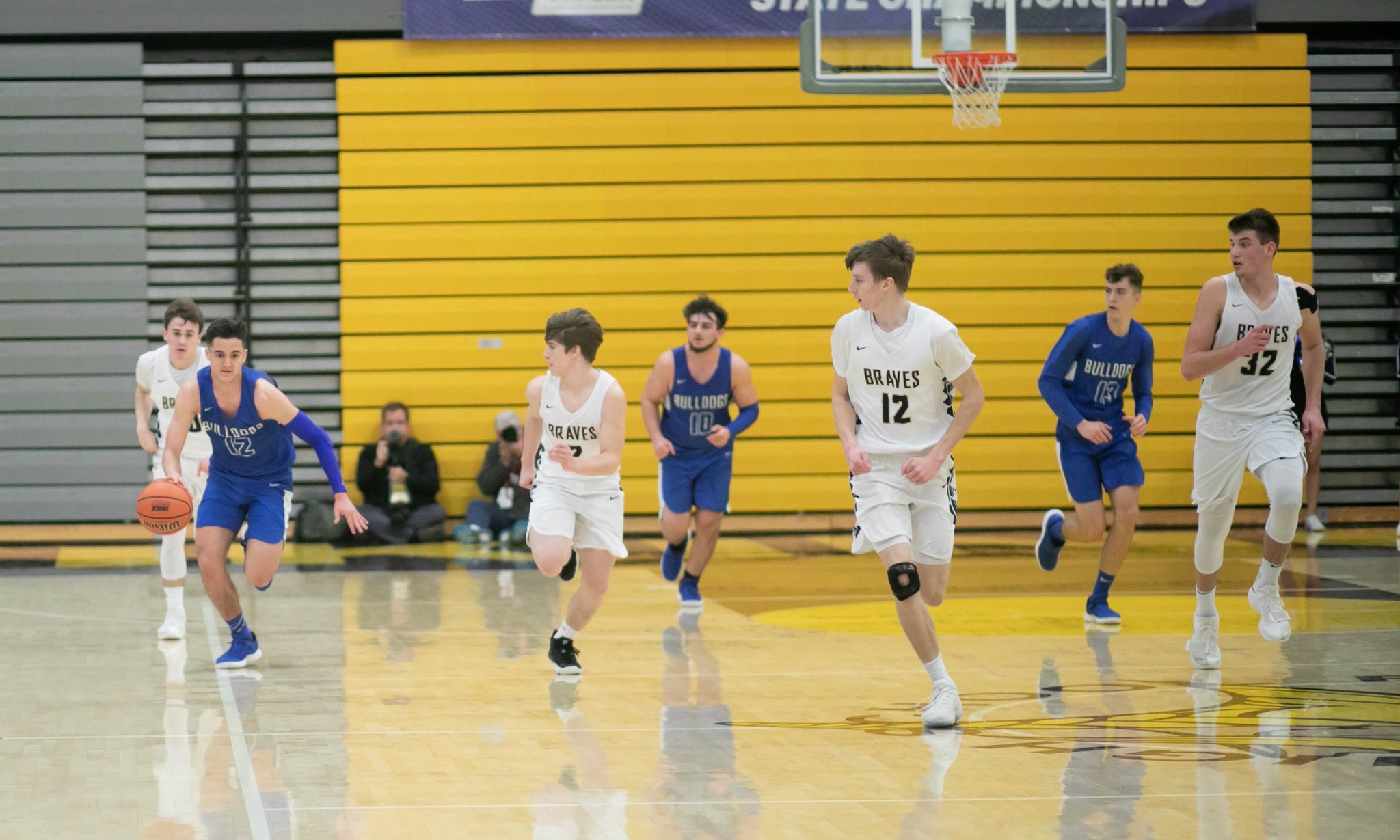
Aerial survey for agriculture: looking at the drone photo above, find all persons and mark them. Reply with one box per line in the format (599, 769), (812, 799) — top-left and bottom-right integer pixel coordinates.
(134, 297), (212, 640)
(1180, 208), (1338, 675)
(831, 233), (985, 726)
(641, 298), (760, 606)
(160, 317), (370, 669)
(518, 308), (628, 672)
(335, 401), (449, 544)
(454, 413), (534, 546)
(1033, 263), (1154, 622)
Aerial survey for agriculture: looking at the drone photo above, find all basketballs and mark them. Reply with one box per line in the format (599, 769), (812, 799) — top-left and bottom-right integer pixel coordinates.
(135, 477), (193, 535)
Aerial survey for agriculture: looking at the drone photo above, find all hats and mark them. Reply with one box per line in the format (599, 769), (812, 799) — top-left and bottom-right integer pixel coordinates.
(494, 412), (519, 429)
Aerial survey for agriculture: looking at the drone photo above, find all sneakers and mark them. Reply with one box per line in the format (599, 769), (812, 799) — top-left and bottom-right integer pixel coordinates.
(1305, 514), (1324, 531)
(560, 550), (578, 580)
(547, 630), (583, 673)
(1085, 596), (1121, 622)
(920, 679), (963, 726)
(216, 631), (262, 668)
(1035, 509), (1063, 571)
(660, 530), (692, 580)
(1189, 612), (1221, 666)
(1248, 585), (1292, 642)
(158, 611), (186, 638)
(678, 578), (701, 605)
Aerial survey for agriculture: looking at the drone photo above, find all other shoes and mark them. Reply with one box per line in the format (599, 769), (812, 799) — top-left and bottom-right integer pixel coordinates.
(417, 528), (433, 539)
(498, 522), (528, 544)
(452, 525), (491, 544)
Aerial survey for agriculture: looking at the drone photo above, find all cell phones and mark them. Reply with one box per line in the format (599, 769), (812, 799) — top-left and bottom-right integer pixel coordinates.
(385, 430), (402, 451)
(499, 425), (519, 445)
